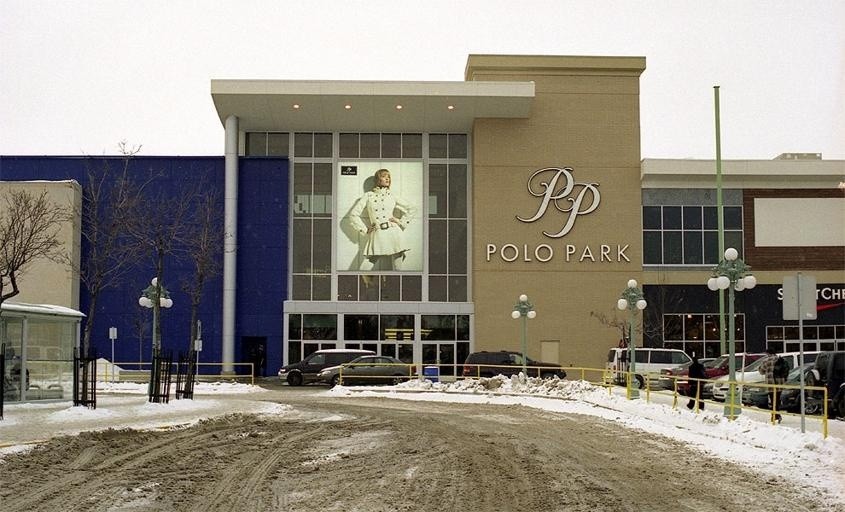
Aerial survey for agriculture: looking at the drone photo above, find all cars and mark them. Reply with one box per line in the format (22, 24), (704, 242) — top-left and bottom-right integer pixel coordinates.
(660, 351), (845, 418)
(318, 356), (417, 386)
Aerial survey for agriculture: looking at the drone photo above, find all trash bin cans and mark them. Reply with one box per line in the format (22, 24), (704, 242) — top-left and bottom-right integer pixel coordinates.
(425, 367), (438, 383)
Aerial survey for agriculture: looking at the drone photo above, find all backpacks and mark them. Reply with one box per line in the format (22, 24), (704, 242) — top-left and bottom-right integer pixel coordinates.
(773, 357), (790, 378)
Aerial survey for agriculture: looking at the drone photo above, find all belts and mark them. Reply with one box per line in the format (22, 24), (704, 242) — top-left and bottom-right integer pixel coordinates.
(373, 222), (397, 230)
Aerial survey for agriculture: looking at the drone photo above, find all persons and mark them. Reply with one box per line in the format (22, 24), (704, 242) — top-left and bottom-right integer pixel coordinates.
(9, 354), (29, 389)
(688, 357), (708, 411)
(347, 168), (420, 271)
(757, 347), (790, 424)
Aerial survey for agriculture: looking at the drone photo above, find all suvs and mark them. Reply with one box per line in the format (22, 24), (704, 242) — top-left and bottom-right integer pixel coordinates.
(278, 349), (375, 386)
(463, 351), (566, 379)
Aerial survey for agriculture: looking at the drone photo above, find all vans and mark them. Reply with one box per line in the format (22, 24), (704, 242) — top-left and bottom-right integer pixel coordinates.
(603, 347), (692, 389)
(6, 345), (64, 374)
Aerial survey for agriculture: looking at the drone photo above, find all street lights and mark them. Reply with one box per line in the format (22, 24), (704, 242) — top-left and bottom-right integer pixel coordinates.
(617, 280), (647, 399)
(708, 248), (756, 416)
(139, 278), (173, 396)
(512, 293), (536, 379)
